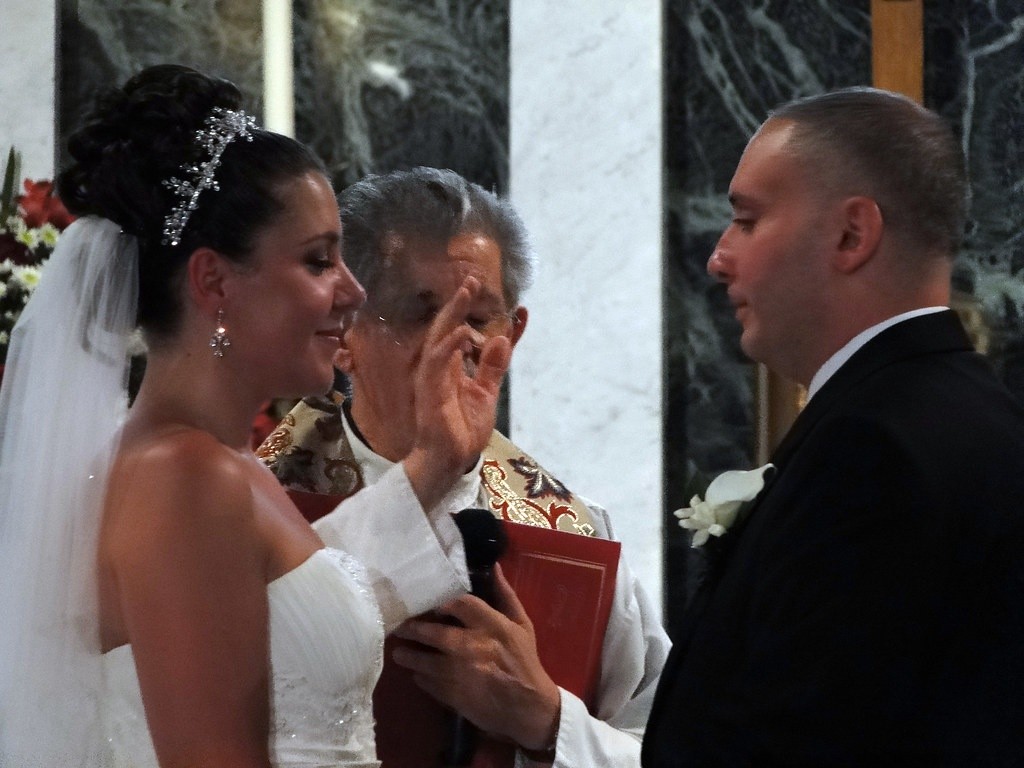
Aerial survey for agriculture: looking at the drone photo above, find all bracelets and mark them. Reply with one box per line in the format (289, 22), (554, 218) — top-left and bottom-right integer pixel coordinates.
(521, 708), (560, 764)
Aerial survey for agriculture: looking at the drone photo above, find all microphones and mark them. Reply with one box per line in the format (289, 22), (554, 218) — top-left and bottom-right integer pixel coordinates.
(437, 507), (508, 768)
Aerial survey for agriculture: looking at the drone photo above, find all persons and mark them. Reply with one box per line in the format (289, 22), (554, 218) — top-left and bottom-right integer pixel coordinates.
(639, 85), (1024, 768)
(0, 61), (673, 768)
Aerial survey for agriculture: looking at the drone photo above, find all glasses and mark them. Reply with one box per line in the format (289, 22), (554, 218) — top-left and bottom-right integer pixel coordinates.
(376, 291), (510, 350)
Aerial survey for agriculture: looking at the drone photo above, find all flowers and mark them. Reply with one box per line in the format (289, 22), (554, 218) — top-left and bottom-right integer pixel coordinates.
(0, 146), (148, 387)
(673, 462), (775, 549)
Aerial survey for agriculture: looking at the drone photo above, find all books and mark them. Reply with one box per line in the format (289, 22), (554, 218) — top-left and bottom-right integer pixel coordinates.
(284, 488), (621, 768)
